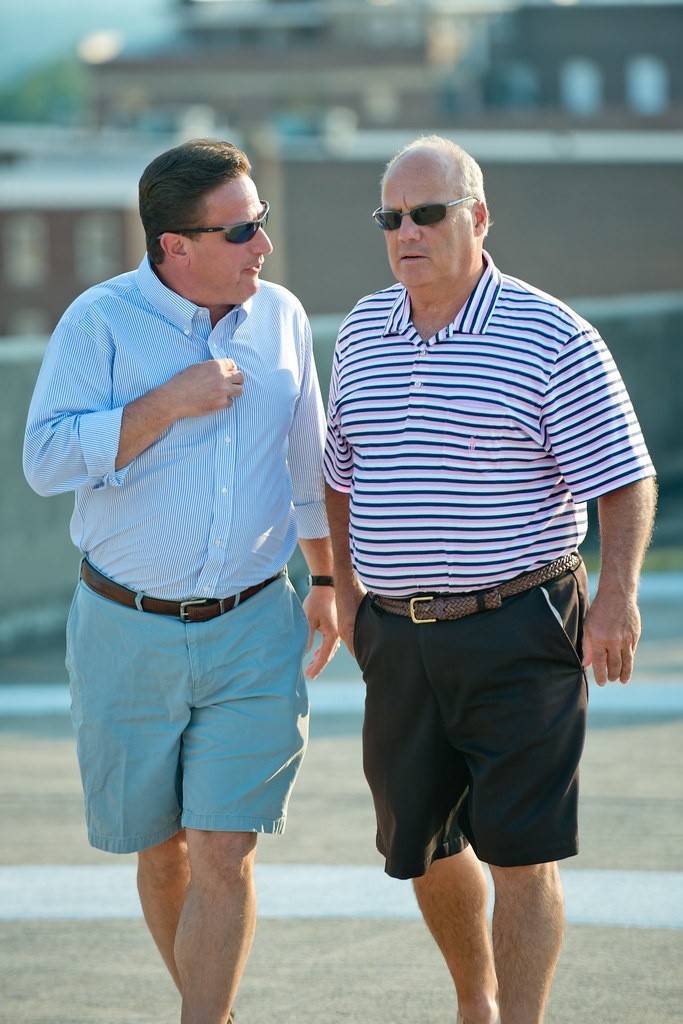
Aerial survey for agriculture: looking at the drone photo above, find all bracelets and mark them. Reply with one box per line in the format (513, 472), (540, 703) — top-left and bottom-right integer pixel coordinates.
(306, 575), (336, 590)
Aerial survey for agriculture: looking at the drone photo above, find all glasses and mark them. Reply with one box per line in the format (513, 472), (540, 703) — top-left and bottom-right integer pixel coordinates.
(155, 200), (270, 244)
(372, 195), (481, 231)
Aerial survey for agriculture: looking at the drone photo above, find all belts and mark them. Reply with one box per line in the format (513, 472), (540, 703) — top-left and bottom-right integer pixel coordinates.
(369, 552), (581, 623)
(81, 557), (280, 623)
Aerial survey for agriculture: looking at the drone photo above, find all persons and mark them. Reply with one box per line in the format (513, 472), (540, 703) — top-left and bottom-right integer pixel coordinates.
(21, 138), (337, 1024)
(321, 136), (658, 1024)
